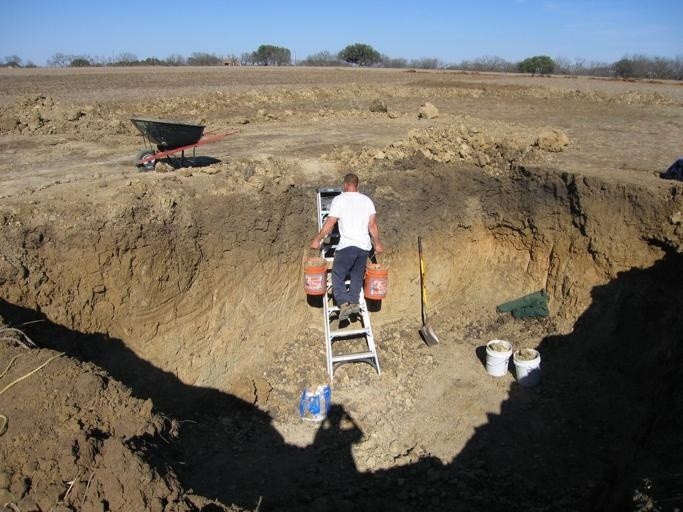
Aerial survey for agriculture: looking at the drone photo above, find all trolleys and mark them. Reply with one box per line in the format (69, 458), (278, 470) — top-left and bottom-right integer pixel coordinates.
(130, 118), (240, 171)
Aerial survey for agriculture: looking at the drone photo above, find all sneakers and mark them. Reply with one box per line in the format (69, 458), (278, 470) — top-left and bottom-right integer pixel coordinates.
(348, 303), (360, 314)
(338, 301), (353, 320)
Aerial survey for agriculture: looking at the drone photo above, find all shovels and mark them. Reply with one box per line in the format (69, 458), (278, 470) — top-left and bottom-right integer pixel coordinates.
(418, 236), (440, 347)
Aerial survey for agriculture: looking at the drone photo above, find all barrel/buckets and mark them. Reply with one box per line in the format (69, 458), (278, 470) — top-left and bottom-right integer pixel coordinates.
(485, 340), (513, 377)
(513, 348), (542, 388)
(363, 252), (389, 300)
(301, 257), (328, 296)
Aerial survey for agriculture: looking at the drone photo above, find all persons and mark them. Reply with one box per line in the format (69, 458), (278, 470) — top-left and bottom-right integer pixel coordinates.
(308, 173), (383, 327)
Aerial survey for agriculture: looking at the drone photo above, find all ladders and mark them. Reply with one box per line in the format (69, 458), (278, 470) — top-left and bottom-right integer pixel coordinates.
(316, 187), (381, 382)
